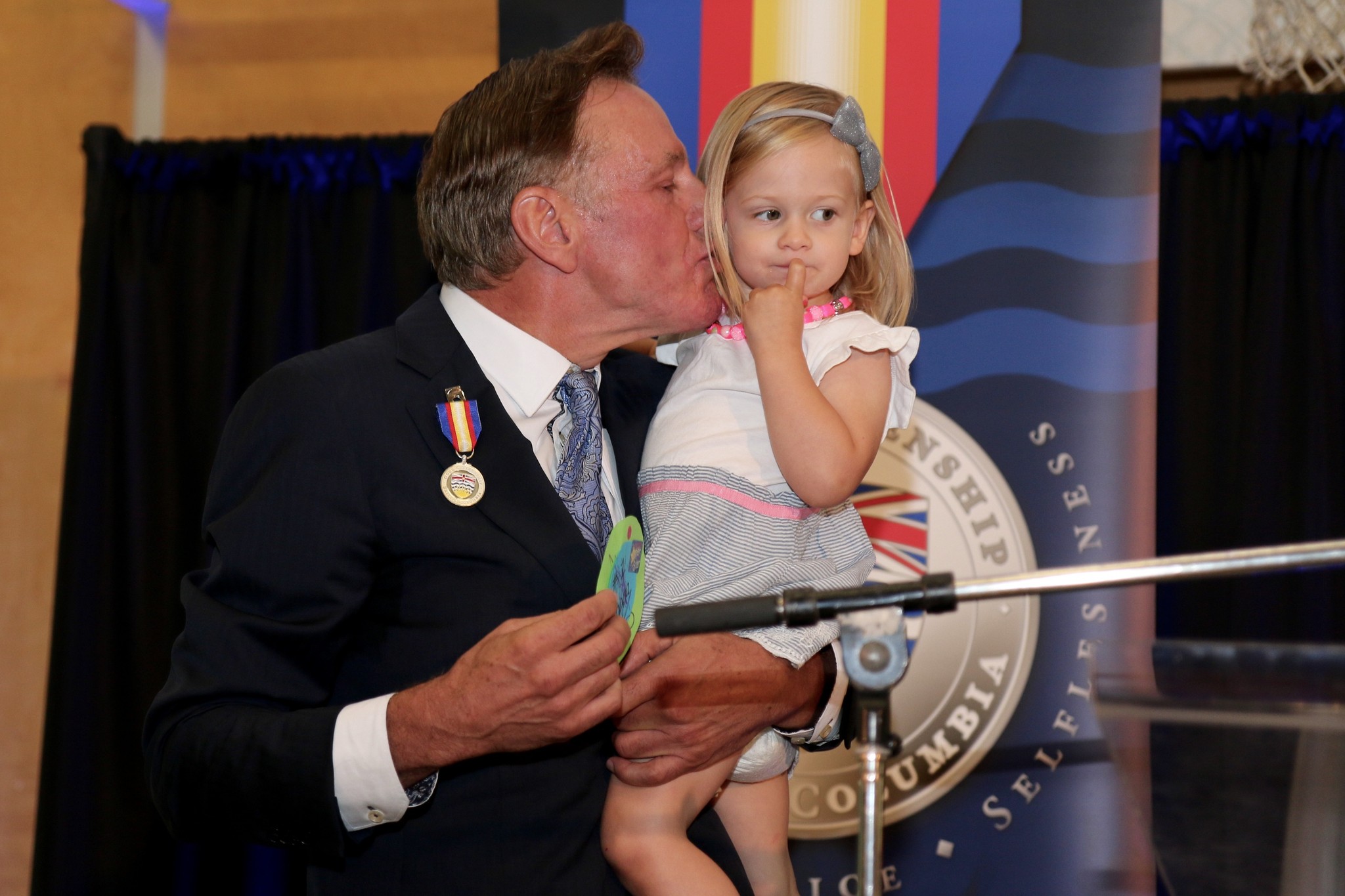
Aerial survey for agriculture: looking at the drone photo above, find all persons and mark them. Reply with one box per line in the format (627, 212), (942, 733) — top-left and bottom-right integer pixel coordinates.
(135, 23), (855, 896)
(594, 83), (919, 896)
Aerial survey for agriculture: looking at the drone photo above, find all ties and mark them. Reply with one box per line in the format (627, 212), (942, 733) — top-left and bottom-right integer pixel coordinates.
(559, 369), (615, 560)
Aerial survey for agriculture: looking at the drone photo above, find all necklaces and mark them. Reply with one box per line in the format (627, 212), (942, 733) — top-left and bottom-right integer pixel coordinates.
(707, 296), (852, 340)
